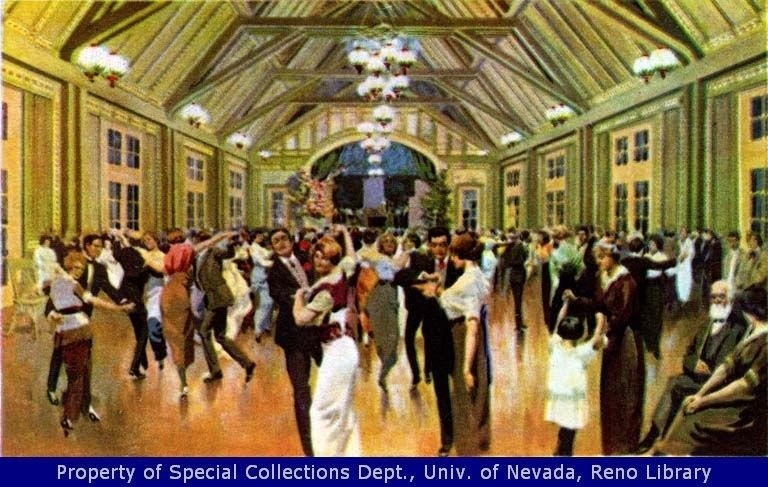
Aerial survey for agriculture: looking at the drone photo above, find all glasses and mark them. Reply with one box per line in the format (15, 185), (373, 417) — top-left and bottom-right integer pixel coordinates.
(382, 239), (396, 243)
(430, 241), (447, 249)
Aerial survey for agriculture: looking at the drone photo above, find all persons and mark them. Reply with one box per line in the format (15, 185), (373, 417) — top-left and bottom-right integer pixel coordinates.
(549, 225), (586, 331)
(57, 238), (82, 266)
(646, 233), (669, 360)
(543, 294), (604, 458)
(142, 228), (231, 400)
(619, 236), (687, 338)
(249, 228), (274, 344)
(533, 230), (552, 325)
(574, 227), (592, 254)
(737, 232), (768, 288)
(647, 287), (768, 458)
(296, 225), (330, 255)
(478, 230), (508, 283)
(491, 229), (530, 331)
(44, 234), (132, 406)
(266, 228), (335, 458)
(118, 247), (164, 380)
(43, 252), (137, 438)
(232, 231), (253, 283)
(220, 261), (253, 341)
(349, 228), (363, 252)
(392, 232), (432, 391)
(436, 232), (493, 458)
(564, 241), (645, 458)
(584, 235), (599, 277)
(674, 224), (696, 308)
(663, 229), (677, 265)
(394, 226), (463, 457)
(690, 226), (702, 281)
(723, 232), (745, 281)
(363, 229), (376, 246)
(239, 226), (253, 245)
(292, 223), (360, 457)
(507, 226), (516, 243)
(191, 230), (257, 384)
(700, 229), (723, 312)
(33, 234), (57, 288)
(361, 234), (414, 395)
(594, 226), (618, 253)
(95, 240), (125, 291)
(116, 230), (166, 371)
(634, 281), (745, 455)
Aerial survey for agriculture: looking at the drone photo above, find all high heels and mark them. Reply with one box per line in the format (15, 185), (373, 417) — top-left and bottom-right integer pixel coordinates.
(255, 334), (261, 344)
(180, 385), (188, 398)
(47, 390), (59, 405)
(62, 420), (72, 437)
(88, 407), (99, 422)
(159, 362), (165, 372)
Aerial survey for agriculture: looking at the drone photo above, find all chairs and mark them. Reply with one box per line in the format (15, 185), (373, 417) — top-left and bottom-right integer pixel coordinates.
(7, 256), (52, 340)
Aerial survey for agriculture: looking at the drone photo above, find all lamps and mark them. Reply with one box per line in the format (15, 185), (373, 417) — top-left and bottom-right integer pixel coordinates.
(501, 132), (522, 148)
(104, 46), (127, 87)
(651, 46), (678, 79)
(546, 106), (556, 126)
(345, 24), (418, 176)
(634, 55), (651, 82)
(77, 44), (108, 82)
(181, 101), (207, 128)
(233, 132), (248, 148)
(556, 103), (571, 123)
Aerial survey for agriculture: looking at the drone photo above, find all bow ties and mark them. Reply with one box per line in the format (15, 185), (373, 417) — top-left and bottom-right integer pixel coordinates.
(85, 258), (95, 265)
(712, 318), (726, 324)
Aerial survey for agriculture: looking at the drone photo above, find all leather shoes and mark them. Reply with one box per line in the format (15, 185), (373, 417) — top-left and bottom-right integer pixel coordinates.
(203, 370), (222, 382)
(440, 445), (452, 456)
(245, 362), (256, 382)
(424, 369), (431, 385)
(379, 380), (388, 392)
(409, 376), (422, 391)
(129, 370), (145, 377)
(637, 434), (658, 453)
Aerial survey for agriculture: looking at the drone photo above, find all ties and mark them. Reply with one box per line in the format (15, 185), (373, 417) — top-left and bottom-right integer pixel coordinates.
(438, 259), (446, 282)
(290, 261), (307, 289)
